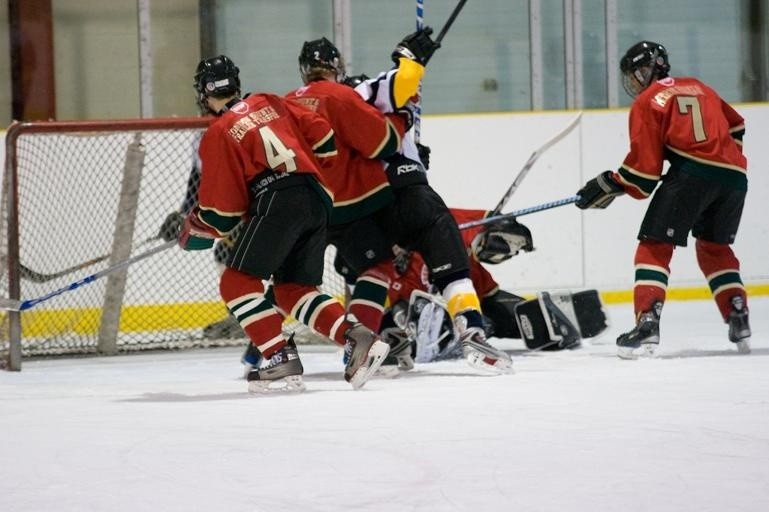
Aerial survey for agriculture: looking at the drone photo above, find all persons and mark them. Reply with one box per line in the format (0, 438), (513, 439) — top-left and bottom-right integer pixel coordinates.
(173, 54), (390, 394)
(383, 204), (550, 340)
(578, 38), (754, 361)
(282, 34), (420, 367)
(340, 26), (515, 370)
(157, 126), (213, 243)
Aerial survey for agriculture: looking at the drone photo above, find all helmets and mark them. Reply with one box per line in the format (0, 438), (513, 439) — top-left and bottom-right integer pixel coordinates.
(344, 74), (369, 88)
(298, 37), (346, 85)
(192, 55), (241, 118)
(620, 41), (670, 99)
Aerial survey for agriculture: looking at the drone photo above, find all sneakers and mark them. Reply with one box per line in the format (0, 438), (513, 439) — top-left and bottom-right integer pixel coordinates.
(616, 300), (663, 347)
(454, 315), (513, 368)
(729, 295), (751, 342)
(343, 312), (380, 382)
(248, 331), (303, 380)
(241, 340), (263, 368)
(378, 327), (411, 357)
(203, 315), (246, 340)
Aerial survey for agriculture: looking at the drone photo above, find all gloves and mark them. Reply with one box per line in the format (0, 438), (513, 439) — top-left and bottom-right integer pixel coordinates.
(575, 170), (625, 210)
(391, 25), (440, 67)
(175, 201), (215, 253)
(160, 210), (184, 242)
